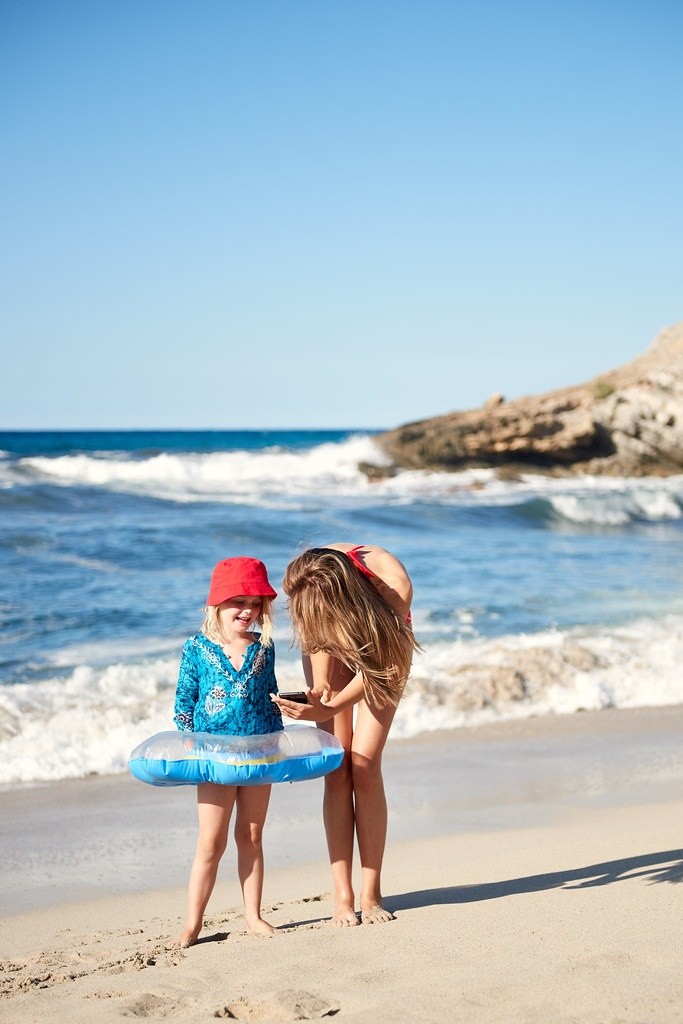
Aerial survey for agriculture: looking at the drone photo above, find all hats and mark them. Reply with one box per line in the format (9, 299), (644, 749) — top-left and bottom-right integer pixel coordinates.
(206, 558), (277, 606)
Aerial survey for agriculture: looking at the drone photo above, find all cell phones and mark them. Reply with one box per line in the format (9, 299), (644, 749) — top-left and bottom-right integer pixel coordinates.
(278, 691), (308, 705)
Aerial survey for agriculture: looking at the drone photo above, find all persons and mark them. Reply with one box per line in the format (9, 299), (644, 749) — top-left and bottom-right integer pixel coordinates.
(269, 542), (415, 929)
(165, 557), (285, 952)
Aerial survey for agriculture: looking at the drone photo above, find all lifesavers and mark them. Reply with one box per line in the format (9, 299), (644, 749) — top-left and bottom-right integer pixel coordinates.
(126, 725), (347, 788)
